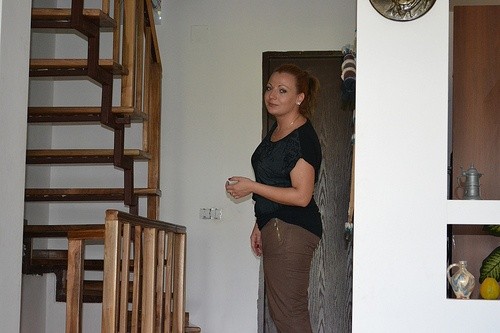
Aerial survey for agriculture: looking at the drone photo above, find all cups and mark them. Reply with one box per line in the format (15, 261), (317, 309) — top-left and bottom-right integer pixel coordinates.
(457, 161), (482, 200)
(447, 261), (476, 299)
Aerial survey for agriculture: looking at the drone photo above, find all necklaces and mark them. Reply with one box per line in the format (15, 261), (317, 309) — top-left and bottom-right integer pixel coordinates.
(274, 115), (300, 134)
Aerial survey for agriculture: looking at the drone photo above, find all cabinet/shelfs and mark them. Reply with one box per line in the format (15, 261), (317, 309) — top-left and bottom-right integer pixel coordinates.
(446, 1), (499, 301)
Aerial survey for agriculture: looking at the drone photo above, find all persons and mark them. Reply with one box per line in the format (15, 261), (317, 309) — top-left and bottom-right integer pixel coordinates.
(223, 63), (322, 333)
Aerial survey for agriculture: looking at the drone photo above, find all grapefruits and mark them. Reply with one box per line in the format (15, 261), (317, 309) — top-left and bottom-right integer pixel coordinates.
(481, 278), (500, 300)
